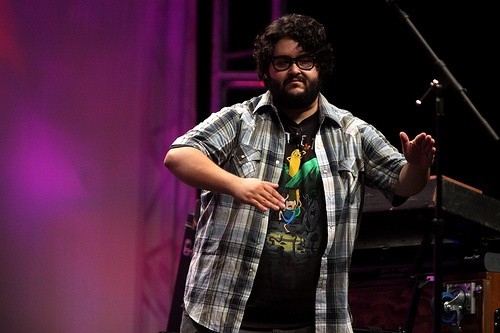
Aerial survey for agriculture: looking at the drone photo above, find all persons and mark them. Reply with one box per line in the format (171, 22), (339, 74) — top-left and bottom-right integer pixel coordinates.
(163, 14), (436, 333)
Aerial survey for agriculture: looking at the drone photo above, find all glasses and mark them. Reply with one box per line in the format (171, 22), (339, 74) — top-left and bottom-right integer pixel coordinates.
(271, 54), (316, 71)
